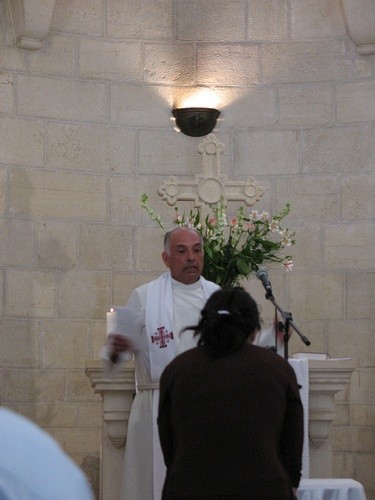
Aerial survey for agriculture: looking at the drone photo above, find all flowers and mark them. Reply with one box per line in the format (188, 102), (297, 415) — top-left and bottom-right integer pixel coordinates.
(139, 192), (296, 289)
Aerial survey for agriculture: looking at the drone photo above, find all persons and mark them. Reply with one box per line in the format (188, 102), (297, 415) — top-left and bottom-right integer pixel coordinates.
(155, 286), (304, 500)
(99, 227), (293, 500)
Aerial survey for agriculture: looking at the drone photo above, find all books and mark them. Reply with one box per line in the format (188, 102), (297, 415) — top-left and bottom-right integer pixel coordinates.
(293, 352), (327, 360)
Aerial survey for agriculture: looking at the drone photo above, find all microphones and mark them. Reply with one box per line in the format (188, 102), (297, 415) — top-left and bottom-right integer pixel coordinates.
(256, 269), (271, 295)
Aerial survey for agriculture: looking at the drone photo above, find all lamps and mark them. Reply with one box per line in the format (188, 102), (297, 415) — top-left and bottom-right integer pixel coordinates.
(172, 107), (220, 138)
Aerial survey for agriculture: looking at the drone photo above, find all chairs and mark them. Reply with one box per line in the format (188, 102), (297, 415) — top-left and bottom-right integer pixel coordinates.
(288, 358), (366, 500)
